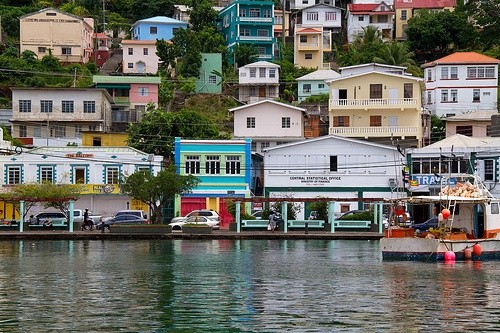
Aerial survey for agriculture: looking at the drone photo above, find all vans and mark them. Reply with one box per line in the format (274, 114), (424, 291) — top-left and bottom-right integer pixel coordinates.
(101, 210), (147, 224)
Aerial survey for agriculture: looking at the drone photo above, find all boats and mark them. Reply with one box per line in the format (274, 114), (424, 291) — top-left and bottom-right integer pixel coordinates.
(380, 195), (500, 263)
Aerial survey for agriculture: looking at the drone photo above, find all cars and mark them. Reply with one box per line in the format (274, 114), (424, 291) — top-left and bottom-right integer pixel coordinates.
(382, 216), (390, 229)
(335, 210), (365, 220)
(251, 211), (282, 221)
(25, 212), (68, 222)
(168, 216), (220, 230)
(392, 212), (413, 228)
(307, 211), (329, 223)
(96, 214), (151, 230)
(409, 218), (437, 233)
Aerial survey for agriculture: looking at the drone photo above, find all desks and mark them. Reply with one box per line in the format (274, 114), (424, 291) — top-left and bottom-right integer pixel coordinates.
(385, 227), (414, 238)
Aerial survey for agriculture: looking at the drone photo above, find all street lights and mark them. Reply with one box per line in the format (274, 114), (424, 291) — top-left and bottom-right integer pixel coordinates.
(147, 154), (154, 224)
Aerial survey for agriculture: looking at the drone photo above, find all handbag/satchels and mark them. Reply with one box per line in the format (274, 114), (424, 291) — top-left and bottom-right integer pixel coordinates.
(273, 217), (277, 221)
(267, 224), (271, 230)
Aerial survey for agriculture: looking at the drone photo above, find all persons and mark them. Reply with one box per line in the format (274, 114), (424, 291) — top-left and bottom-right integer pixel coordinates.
(10, 215), (17, 231)
(29, 214), (37, 229)
(81, 208), (93, 230)
(43, 215), (52, 229)
(268, 211), (277, 233)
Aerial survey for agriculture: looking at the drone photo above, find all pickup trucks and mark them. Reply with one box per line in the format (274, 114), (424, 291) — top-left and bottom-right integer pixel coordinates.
(61, 209), (102, 225)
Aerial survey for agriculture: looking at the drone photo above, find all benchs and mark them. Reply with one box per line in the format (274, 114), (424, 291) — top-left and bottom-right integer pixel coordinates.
(241, 220), (270, 229)
(0, 218), (18, 230)
(288, 220), (325, 230)
(334, 221), (372, 231)
(29, 217), (67, 230)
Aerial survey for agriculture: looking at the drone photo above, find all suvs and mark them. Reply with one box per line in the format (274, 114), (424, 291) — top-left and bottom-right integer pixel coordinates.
(184, 209), (221, 223)
